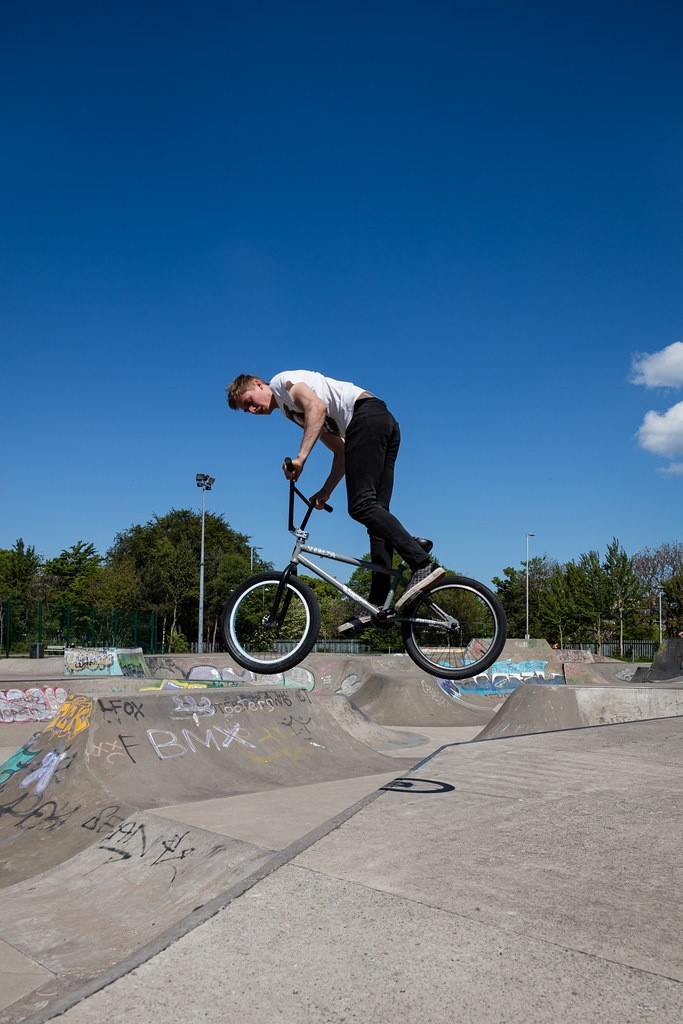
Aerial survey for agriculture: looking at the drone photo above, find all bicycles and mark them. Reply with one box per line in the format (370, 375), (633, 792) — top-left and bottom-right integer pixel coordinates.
(222, 457), (509, 683)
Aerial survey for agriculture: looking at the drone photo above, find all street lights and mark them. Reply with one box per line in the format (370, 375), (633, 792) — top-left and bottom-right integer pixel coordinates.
(656, 586), (667, 647)
(525, 532), (536, 639)
(195, 471), (215, 650)
(249, 546), (263, 574)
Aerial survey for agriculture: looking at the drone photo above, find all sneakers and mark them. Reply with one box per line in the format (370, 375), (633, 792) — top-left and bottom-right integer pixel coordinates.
(336, 603), (384, 633)
(395, 562), (446, 612)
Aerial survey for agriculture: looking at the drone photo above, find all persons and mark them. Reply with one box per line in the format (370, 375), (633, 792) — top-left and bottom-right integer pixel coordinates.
(70, 642), (76, 648)
(62, 641), (67, 655)
(227, 369), (447, 634)
